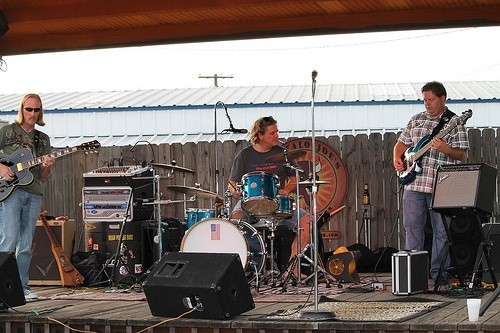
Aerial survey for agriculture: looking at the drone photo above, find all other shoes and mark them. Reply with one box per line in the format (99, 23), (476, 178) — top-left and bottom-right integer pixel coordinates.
(437, 277), (452, 291)
(24, 290), (38, 301)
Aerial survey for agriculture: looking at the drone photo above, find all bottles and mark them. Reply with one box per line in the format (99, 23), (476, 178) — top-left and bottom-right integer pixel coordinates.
(362, 183), (370, 205)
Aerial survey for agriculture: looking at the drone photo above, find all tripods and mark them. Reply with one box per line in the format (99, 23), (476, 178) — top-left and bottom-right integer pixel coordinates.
(253, 164), (343, 293)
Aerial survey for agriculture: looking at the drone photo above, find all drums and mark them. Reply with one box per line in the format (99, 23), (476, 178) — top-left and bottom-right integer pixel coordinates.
(184, 208), (219, 229)
(179, 217), (266, 284)
(253, 195), (297, 217)
(241, 172), (280, 214)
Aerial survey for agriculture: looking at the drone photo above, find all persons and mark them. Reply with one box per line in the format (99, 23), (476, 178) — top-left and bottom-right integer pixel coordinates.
(228, 117), (301, 282)
(394, 81), (469, 286)
(0, 93), (55, 299)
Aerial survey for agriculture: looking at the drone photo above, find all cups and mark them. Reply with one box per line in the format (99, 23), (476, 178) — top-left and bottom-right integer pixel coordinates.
(467, 298), (481, 321)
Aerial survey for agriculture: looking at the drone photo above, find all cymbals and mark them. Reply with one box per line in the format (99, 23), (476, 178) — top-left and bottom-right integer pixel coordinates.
(290, 180), (331, 185)
(167, 184), (220, 200)
(153, 163), (195, 173)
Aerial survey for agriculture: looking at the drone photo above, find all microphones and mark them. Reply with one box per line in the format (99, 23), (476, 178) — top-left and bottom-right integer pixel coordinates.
(223, 129), (248, 133)
(312, 70), (317, 81)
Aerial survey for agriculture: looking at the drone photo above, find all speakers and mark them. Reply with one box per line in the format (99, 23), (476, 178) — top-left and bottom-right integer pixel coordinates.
(445, 211), (487, 286)
(426, 161), (497, 215)
(0, 251), (28, 309)
(20, 218), (76, 286)
(103, 219), (157, 287)
(143, 252), (257, 321)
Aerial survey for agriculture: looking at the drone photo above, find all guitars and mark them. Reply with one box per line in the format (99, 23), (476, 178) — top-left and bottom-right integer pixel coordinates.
(0, 140), (101, 203)
(395, 108), (474, 184)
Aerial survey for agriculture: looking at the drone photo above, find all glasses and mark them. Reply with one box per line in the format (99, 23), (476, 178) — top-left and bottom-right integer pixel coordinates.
(22, 106), (41, 112)
